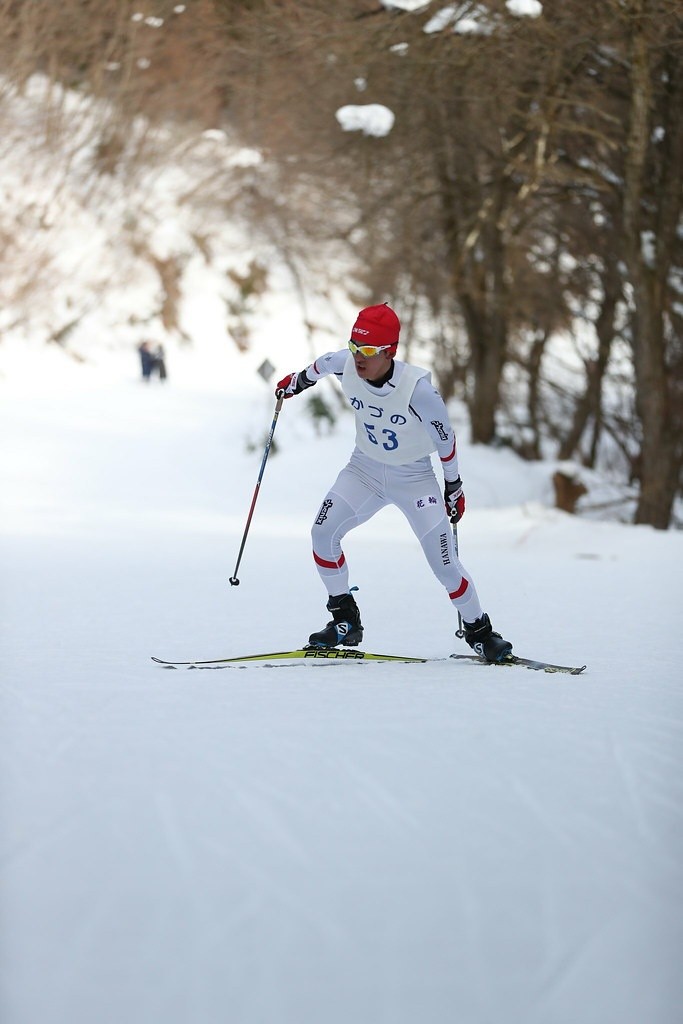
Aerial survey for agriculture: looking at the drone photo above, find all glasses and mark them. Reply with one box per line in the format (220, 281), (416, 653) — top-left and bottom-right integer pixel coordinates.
(348, 339), (393, 357)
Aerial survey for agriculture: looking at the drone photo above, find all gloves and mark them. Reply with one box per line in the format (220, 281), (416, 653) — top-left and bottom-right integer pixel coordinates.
(444, 474), (465, 523)
(275, 370), (317, 398)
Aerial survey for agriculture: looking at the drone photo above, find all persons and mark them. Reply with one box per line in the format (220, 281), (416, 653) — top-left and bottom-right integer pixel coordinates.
(140, 340), (166, 378)
(274, 302), (512, 662)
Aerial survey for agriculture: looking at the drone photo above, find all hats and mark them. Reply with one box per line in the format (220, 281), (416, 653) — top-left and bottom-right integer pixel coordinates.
(351, 301), (400, 356)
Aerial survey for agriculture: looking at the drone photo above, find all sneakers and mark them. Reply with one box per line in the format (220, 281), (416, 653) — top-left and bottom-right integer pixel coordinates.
(309, 587), (363, 648)
(463, 611), (513, 662)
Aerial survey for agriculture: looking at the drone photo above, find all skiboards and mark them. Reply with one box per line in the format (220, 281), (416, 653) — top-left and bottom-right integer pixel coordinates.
(150, 647), (588, 682)
